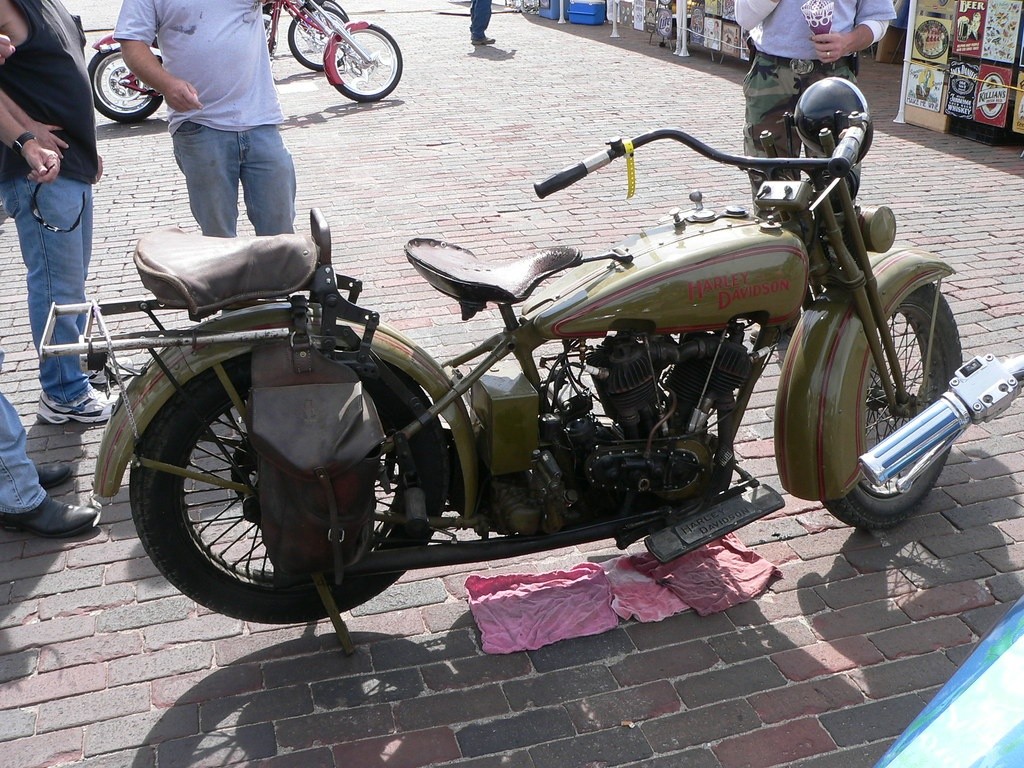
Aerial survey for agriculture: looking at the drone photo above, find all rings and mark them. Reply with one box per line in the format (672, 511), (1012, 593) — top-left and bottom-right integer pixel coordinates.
(827, 51), (831, 58)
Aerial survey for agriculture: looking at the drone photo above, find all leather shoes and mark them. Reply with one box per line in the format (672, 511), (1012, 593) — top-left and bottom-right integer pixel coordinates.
(34, 462), (72, 489)
(0, 494), (98, 538)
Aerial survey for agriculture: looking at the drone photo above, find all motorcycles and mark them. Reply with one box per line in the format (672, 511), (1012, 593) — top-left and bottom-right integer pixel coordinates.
(32, 72), (964, 660)
(87, 0), (404, 124)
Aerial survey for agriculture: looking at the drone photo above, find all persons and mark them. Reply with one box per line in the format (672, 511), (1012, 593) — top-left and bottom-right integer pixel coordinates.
(0, 0), (133, 424)
(113, 0), (296, 239)
(0, 35), (99, 536)
(734, 0), (896, 341)
(470, 0), (496, 45)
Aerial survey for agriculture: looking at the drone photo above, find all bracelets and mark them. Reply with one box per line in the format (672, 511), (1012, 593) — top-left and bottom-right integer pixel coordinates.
(13, 131), (36, 158)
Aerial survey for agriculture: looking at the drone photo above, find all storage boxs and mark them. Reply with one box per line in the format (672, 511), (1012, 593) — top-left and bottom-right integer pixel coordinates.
(539, 0), (570, 20)
(567, 0), (605, 25)
(950, 100), (1024, 145)
(904, 84), (950, 133)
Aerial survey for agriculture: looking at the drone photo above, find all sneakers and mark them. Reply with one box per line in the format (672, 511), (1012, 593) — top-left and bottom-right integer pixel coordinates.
(36, 387), (120, 424)
(78, 353), (134, 383)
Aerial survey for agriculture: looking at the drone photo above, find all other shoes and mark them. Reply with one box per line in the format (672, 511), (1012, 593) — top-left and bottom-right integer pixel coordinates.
(471, 37), (495, 45)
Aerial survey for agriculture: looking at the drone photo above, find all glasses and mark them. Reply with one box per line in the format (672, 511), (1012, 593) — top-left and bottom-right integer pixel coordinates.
(29, 164), (85, 233)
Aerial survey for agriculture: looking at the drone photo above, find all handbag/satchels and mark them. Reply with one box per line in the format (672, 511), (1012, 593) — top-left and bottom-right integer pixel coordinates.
(249, 340), (383, 574)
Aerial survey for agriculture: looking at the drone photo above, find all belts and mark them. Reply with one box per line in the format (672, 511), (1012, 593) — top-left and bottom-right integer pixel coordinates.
(763, 51), (855, 74)
